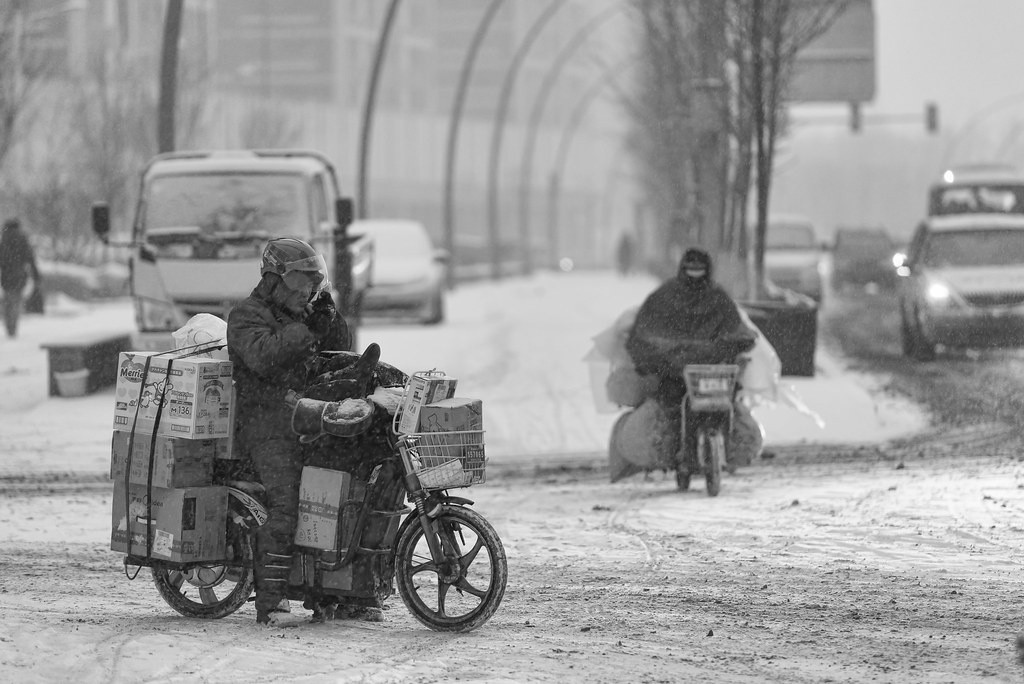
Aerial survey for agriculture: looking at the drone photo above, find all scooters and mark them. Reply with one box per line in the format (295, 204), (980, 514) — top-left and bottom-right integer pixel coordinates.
(653, 331), (752, 497)
(123, 344), (507, 633)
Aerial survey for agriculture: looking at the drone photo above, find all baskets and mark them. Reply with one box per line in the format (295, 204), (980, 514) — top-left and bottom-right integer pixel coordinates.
(681, 363), (740, 413)
(393, 368), (486, 492)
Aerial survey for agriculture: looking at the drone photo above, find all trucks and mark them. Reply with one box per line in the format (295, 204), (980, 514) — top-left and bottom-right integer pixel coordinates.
(92, 149), (373, 353)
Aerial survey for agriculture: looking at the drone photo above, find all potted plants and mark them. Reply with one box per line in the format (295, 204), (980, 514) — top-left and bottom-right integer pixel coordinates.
(53, 353), (93, 396)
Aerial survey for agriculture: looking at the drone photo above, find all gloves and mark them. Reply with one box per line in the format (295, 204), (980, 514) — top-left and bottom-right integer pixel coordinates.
(292, 397), (375, 444)
(304, 291), (337, 340)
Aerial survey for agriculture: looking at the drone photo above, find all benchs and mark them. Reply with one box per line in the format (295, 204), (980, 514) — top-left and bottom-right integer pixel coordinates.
(41, 326), (137, 394)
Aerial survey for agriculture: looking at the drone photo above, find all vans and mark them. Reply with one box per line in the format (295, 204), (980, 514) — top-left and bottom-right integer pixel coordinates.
(927, 165), (1024, 214)
(749, 215), (821, 303)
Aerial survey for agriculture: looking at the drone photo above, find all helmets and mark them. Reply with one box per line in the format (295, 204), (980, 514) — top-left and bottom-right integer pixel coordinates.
(260, 235), (323, 277)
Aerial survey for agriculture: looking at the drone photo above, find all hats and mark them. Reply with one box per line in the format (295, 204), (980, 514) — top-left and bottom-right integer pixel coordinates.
(681, 248), (709, 270)
(5, 215), (23, 227)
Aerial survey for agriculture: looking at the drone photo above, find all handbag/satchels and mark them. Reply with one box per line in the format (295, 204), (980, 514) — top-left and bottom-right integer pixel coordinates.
(23, 286), (43, 314)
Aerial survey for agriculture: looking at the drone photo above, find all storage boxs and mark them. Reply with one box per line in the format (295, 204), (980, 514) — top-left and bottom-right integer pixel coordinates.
(111, 431), (214, 487)
(295, 465), (368, 549)
(109, 480), (227, 563)
(113, 351), (232, 440)
(421, 398), (483, 474)
(398, 376), (458, 434)
(291, 547), (391, 590)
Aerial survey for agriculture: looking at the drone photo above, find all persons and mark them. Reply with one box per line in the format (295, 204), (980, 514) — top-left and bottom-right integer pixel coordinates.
(0, 217), (45, 339)
(227, 238), (390, 627)
(624, 248), (760, 472)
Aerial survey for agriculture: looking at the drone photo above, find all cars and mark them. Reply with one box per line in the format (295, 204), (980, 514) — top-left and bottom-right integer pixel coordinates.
(823, 228), (904, 293)
(898, 212), (1024, 361)
(357, 219), (451, 324)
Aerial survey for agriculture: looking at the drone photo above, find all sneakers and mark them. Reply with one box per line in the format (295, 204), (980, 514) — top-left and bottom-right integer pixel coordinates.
(335, 604), (385, 621)
(257, 610), (300, 629)
